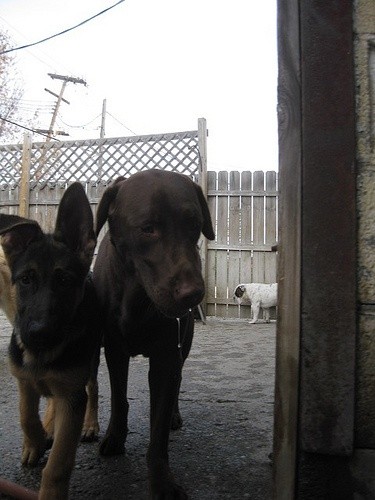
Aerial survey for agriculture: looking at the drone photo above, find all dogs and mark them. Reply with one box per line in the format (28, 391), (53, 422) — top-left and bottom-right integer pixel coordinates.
(93, 168), (215, 500)
(233, 283), (278, 323)
(0, 182), (98, 500)
